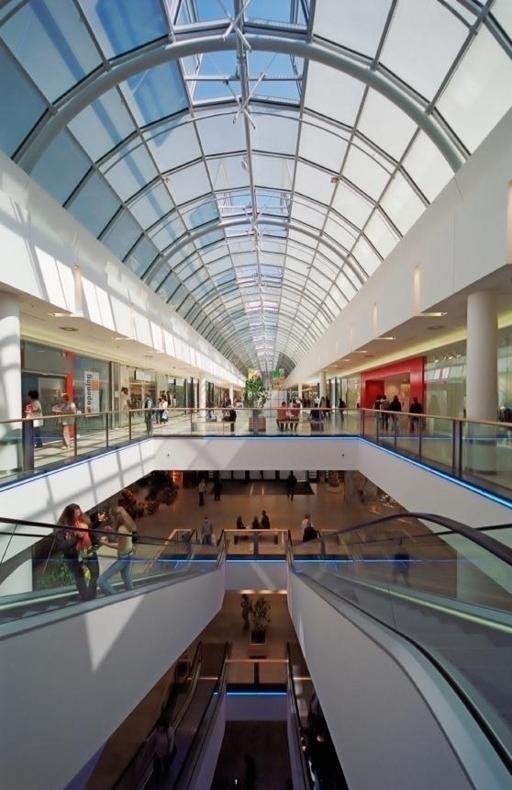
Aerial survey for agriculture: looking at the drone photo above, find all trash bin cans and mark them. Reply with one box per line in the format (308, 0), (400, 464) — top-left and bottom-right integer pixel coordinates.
(0, 439), (19, 474)
(176, 656), (191, 689)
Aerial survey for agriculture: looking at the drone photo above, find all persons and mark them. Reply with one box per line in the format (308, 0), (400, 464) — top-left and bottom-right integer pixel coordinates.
(241, 594), (252, 630)
(141, 658), (333, 789)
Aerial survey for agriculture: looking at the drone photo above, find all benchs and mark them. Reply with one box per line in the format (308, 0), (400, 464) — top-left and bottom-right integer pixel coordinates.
(190, 421), (236, 434)
(278, 419), (325, 432)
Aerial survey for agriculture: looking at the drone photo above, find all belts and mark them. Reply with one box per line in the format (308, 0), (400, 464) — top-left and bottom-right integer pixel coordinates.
(119, 550), (134, 558)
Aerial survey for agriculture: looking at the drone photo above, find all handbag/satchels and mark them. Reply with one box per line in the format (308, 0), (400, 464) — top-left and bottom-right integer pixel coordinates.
(132, 532), (139, 544)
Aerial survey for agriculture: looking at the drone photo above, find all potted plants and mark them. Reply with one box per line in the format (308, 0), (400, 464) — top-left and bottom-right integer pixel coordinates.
(247, 596), (273, 657)
(243, 373), (273, 433)
